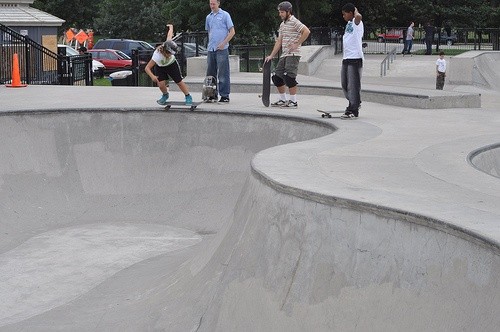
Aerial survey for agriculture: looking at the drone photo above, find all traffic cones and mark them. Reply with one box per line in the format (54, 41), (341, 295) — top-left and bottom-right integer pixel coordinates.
(5, 53), (27, 87)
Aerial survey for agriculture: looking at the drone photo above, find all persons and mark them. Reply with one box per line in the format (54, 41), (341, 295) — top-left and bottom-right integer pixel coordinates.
(145, 24), (192, 105)
(205, 0), (235, 103)
(424, 24), (434, 55)
(341, 2), (364, 120)
(402, 21), (416, 56)
(264, 2), (310, 110)
(436, 52), (446, 90)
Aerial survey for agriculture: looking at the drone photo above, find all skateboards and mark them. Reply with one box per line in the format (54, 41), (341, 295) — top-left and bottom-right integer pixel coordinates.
(317, 104), (361, 118)
(157, 100), (204, 111)
(202, 76), (218, 103)
(259, 59), (272, 107)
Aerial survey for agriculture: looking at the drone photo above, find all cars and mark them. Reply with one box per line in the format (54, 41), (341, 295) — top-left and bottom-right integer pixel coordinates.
(149, 42), (208, 58)
(420, 30), (459, 46)
(375, 28), (405, 43)
(86, 49), (146, 72)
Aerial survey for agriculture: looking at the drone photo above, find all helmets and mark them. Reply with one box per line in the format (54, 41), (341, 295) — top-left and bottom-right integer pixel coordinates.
(278, 2), (292, 12)
(164, 40), (179, 55)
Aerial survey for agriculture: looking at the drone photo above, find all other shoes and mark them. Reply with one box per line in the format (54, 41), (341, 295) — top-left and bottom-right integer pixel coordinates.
(340, 105), (362, 120)
(280, 100), (298, 109)
(271, 100), (288, 107)
(208, 95), (218, 101)
(185, 95), (192, 106)
(219, 96), (229, 104)
(158, 93), (169, 104)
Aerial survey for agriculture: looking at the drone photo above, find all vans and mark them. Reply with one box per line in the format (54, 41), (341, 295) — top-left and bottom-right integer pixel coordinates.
(92, 38), (155, 62)
(57, 45), (106, 78)
